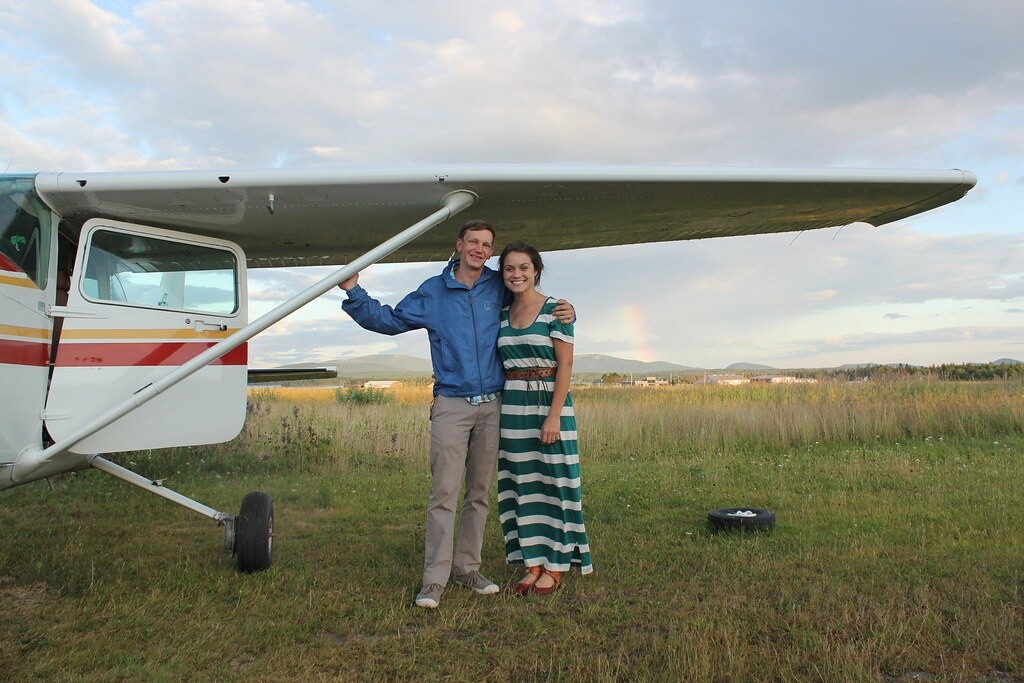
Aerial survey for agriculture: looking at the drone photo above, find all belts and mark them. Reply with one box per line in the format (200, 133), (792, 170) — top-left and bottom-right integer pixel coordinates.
(505, 367), (563, 407)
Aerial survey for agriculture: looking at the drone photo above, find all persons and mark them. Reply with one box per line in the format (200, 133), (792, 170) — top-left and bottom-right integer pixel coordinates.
(431, 242), (595, 596)
(337, 219), (578, 607)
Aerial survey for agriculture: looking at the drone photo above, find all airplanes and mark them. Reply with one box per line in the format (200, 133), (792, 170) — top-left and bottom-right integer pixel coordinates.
(0, 162), (975, 574)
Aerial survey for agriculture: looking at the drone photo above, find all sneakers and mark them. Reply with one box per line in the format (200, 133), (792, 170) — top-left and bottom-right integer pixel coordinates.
(415, 583), (444, 607)
(454, 572), (500, 594)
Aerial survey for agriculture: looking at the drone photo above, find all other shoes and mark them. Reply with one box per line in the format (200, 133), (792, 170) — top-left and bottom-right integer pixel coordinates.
(530, 569), (565, 595)
(510, 567), (540, 596)
